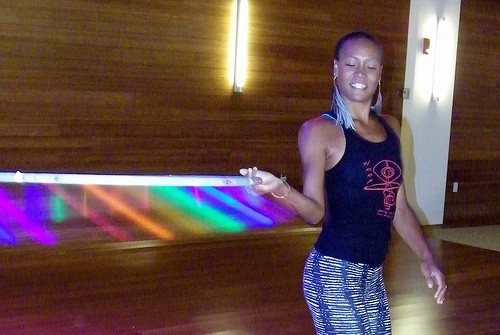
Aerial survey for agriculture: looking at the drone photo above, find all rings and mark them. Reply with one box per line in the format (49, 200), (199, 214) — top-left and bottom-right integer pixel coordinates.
(444, 284), (447, 288)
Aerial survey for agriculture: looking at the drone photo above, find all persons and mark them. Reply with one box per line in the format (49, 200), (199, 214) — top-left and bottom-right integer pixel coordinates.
(239, 32), (448, 335)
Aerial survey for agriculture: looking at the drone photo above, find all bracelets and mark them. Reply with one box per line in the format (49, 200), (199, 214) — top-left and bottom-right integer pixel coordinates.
(270, 173), (290, 200)
